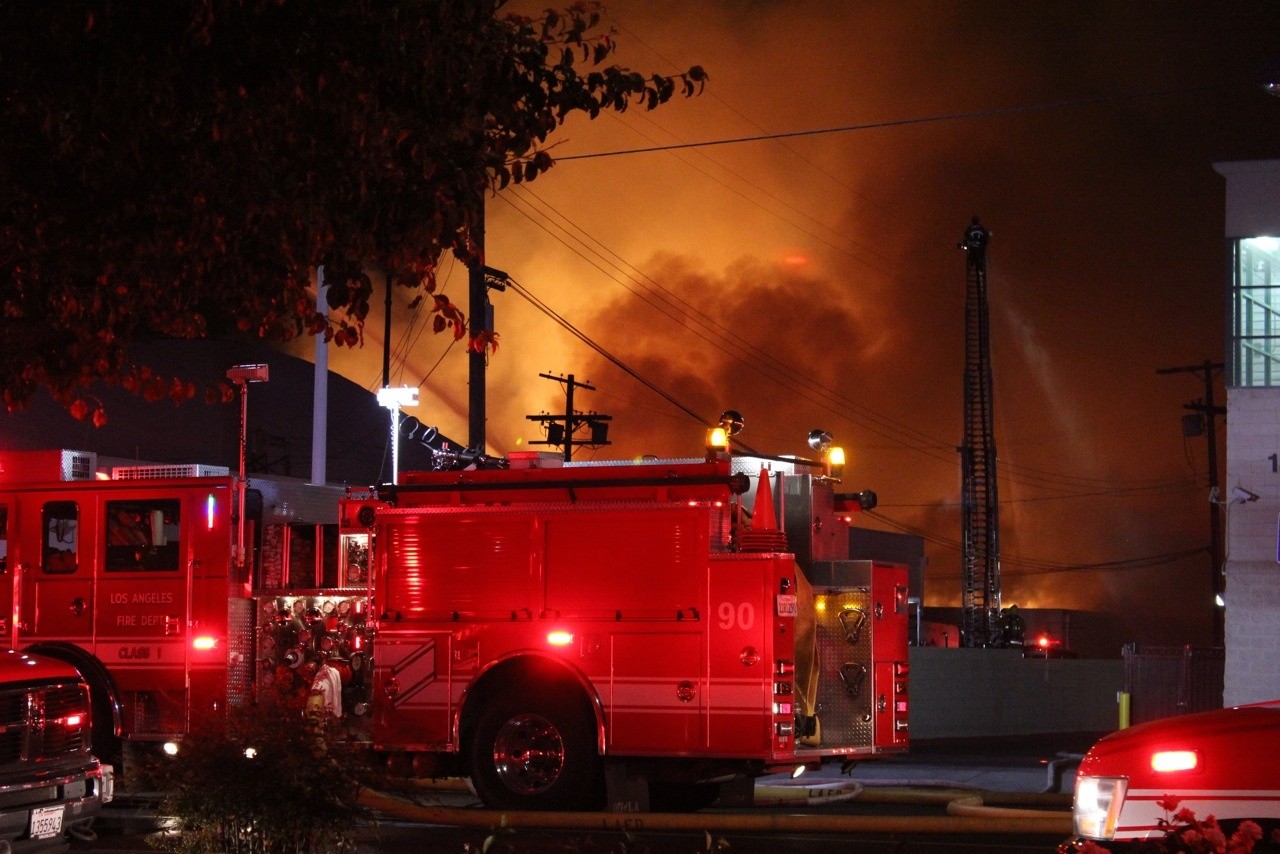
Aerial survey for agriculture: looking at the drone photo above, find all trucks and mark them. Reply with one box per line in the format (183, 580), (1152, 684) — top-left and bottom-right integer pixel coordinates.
(0, 650), (114, 854)
(1059, 700), (1280, 854)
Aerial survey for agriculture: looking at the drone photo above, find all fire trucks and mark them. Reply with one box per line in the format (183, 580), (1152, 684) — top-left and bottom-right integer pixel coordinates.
(1, 363), (926, 813)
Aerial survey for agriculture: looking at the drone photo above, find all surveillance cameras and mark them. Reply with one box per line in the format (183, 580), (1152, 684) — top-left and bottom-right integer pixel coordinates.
(1207, 487), (1220, 503)
(1231, 487), (1253, 501)
